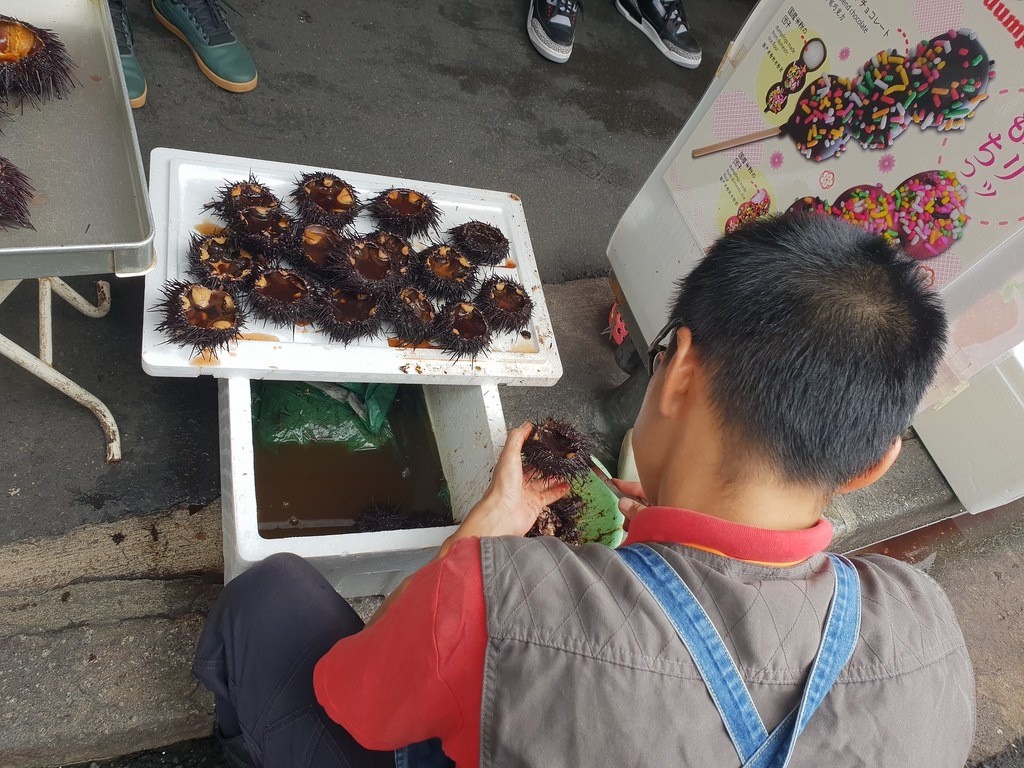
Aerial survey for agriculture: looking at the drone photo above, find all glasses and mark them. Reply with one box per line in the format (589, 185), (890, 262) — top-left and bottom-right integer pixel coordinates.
(646, 317), (690, 381)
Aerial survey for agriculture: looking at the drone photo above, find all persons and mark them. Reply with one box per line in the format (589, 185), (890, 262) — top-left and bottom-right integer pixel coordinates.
(106, 0), (259, 108)
(526, 0), (703, 70)
(192, 213), (978, 767)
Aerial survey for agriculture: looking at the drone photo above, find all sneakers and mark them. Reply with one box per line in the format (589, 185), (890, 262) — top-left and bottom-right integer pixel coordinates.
(151, 0), (258, 93)
(108, 0), (147, 108)
(527, 0), (585, 63)
(615, 0), (702, 69)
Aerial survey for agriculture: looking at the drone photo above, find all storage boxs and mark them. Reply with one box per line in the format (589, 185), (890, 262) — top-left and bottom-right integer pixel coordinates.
(140, 147), (567, 601)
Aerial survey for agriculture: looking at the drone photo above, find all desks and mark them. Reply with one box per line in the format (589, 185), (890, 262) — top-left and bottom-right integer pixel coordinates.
(0, 0), (158, 465)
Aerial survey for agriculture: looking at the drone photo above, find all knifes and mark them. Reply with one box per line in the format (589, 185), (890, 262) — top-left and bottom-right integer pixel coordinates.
(580, 450), (647, 510)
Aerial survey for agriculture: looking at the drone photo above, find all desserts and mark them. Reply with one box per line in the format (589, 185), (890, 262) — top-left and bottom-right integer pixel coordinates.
(783, 168), (970, 261)
(784, 28), (992, 161)
(725, 188), (771, 233)
